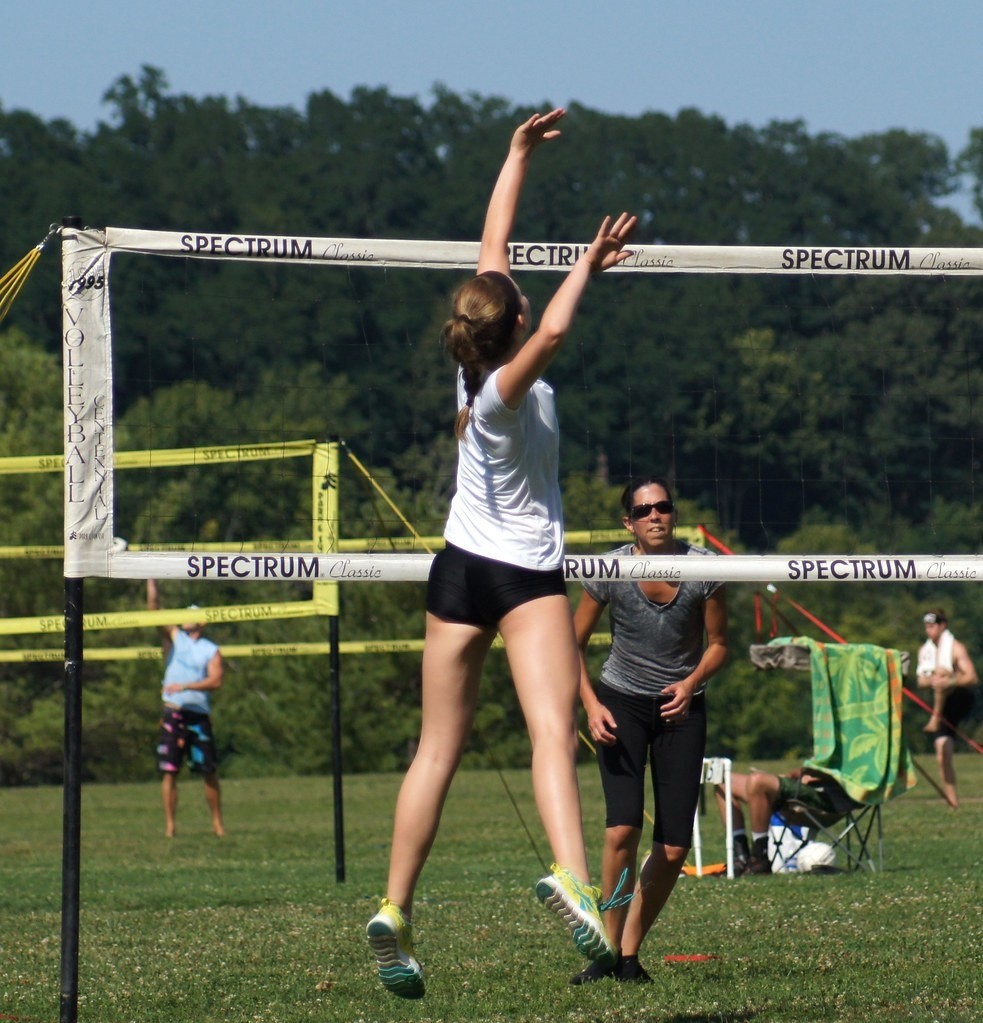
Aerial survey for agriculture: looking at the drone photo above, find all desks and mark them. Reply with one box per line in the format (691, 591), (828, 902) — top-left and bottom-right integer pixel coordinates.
(639, 758), (736, 881)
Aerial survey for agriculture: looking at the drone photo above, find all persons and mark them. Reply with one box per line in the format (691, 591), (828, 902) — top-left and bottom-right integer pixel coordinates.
(366, 107), (637, 999)
(147, 578), (226, 837)
(571, 476), (729, 985)
(915, 608), (983, 809)
(716, 769), (864, 877)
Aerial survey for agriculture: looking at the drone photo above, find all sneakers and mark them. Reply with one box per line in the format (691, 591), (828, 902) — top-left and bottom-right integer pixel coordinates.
(366, 898), (425, 999)
(536, 863), (635, 968)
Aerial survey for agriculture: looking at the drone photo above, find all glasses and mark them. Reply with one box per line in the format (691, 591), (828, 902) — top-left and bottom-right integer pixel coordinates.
(631, 501), (674, 519)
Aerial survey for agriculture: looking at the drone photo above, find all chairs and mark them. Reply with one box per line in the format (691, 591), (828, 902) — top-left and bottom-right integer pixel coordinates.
(770, 798), (883, 877)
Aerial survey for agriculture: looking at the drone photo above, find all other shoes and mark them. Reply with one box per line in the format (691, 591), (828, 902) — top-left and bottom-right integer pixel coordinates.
(618, 955), (656, 984)
(571, 948), (623, 985)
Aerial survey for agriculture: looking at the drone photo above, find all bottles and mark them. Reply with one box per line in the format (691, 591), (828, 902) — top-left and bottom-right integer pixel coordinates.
(786, 843), (797, 872)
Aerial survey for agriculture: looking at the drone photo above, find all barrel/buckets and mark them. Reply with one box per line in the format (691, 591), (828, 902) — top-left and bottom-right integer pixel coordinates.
(768, 812), (810, 874)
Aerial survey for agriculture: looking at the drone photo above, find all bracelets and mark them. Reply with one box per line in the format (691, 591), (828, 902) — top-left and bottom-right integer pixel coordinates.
(181, 684), (187, 690)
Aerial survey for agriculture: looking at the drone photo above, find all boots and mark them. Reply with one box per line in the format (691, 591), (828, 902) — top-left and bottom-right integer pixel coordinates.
(724, 834), (750, 877)
(740, 836), (769, 877)
